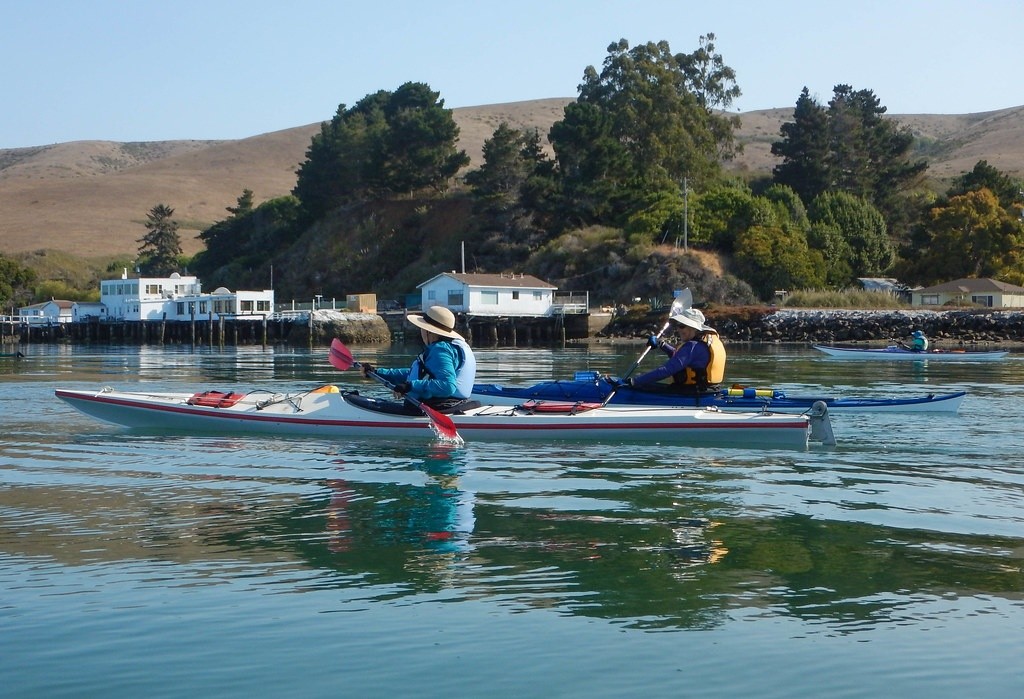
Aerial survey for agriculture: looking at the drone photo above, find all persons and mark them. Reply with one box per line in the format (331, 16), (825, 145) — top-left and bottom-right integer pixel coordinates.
(616, 309), (726, 389)
(359, 306), (476, 403)
(898, 331), (928, 350)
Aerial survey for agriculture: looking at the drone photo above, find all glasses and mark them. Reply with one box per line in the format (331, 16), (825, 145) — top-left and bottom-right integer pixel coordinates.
(674, 322), (686, 329)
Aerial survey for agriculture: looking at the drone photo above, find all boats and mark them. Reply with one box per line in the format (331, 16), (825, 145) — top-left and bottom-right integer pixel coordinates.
(55, 385), (835, 449)
(813, 345), (1012, 361)
(469, 370), (968, 414)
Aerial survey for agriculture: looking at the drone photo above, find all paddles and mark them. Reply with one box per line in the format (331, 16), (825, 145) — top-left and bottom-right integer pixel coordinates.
(328, 337), (456, 439)
(601, 289), (692, 407)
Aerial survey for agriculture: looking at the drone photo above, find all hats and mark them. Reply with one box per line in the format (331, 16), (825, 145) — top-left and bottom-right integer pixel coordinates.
(669, 309), (716, 336)
(407, 306), (463, 340)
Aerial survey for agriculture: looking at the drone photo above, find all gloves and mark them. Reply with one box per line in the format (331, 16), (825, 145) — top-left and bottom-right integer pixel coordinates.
(614, 378), (633, 390)
(646, 336), (664, 350)
